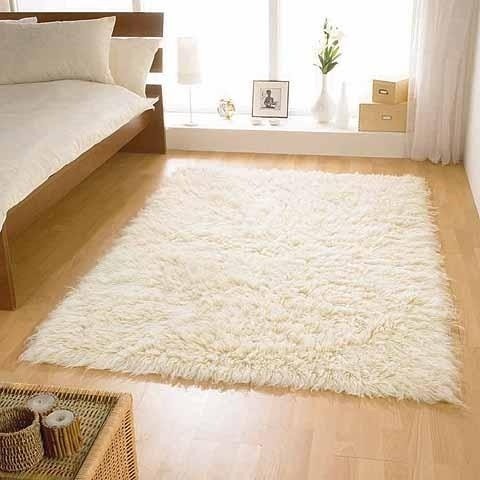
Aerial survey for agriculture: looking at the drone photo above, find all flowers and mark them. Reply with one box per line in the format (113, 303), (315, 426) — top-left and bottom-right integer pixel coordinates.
(313, 17), (343, 74)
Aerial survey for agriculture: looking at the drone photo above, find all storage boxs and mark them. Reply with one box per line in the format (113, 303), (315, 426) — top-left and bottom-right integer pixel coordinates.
(372, 75), (408, 104)
(359, 103), (407, 133)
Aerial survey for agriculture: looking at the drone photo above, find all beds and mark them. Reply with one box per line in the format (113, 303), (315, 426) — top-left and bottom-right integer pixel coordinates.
(0, 12), (166, 310)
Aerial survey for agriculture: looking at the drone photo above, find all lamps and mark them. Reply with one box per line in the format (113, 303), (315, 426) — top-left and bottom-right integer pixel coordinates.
(177, 35), (200, 126)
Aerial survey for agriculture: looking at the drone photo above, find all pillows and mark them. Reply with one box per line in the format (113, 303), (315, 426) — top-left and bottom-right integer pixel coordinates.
(0, 16), (160, 99)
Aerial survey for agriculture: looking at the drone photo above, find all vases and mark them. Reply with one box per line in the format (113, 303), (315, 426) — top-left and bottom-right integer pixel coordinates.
(313, 78), (335, 126)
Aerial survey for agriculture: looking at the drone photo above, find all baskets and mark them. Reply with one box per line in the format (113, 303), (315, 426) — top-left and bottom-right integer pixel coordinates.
(0, 406), (45, 472)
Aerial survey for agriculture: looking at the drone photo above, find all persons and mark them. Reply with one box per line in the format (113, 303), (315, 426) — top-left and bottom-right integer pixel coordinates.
(263, 89), (278, 108)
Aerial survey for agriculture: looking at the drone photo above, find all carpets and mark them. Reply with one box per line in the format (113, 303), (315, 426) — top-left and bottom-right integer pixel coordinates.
(16, 170), (465, 404)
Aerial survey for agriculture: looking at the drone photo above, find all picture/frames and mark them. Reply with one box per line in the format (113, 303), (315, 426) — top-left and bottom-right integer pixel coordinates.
(335, 82), (351, 128)
(251, 80), (290, 119)
